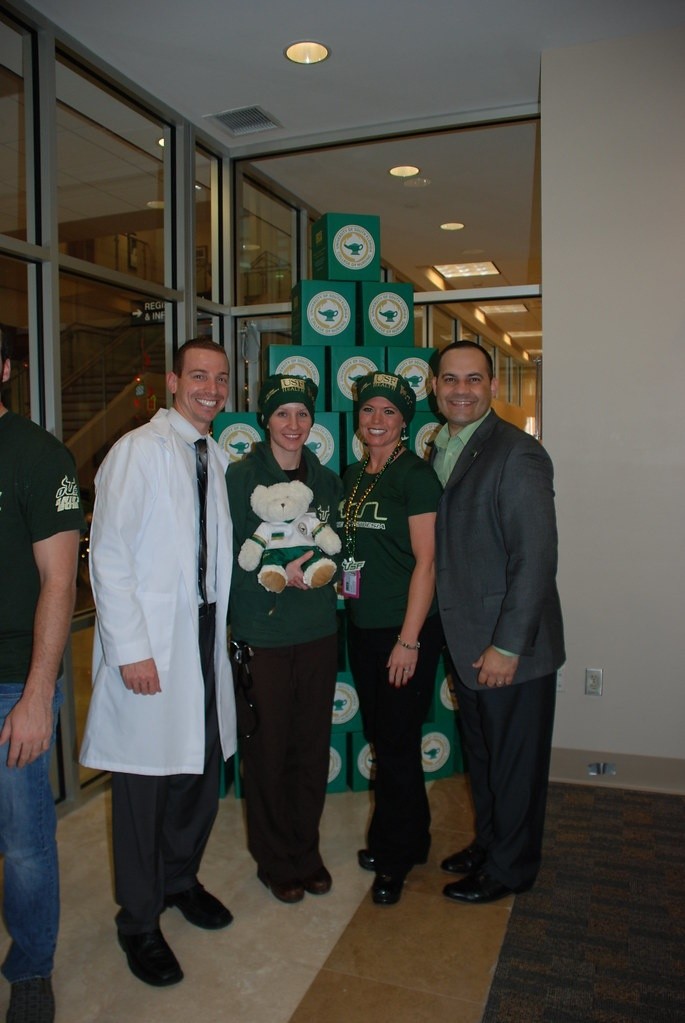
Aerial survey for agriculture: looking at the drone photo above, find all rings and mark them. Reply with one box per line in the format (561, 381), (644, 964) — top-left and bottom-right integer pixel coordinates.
(403, 670), (409, 673)
(300, 584), (304, 587)
(497, 681), (504, 685)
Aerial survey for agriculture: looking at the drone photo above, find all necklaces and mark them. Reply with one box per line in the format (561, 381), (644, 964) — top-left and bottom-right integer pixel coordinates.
(346, 442), (403, 557)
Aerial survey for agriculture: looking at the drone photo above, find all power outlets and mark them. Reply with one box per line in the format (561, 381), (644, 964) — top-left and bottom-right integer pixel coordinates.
(586, 669), (603, 696)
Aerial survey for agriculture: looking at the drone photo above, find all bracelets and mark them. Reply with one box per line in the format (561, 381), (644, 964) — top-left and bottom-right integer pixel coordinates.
(398, 634), (421, 650)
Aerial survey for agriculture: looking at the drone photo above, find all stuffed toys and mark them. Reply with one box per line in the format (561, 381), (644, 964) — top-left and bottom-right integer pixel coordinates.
(238, 480), (342, 593)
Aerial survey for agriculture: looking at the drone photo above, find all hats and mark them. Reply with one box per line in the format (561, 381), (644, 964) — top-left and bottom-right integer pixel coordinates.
(258, 375), (318, 426)
(356, 372), (416, 428)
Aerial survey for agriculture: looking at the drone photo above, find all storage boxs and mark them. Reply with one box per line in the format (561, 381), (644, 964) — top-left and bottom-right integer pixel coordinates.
(201, 213), (463, 795)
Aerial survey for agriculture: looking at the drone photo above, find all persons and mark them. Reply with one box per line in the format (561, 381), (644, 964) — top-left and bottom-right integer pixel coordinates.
(342, 372), (444, 904)
(78, 338), (236, 986)
(225, 374), (344, 904)
(424, 339), (566, 904)
(0, 322), (79, 1023)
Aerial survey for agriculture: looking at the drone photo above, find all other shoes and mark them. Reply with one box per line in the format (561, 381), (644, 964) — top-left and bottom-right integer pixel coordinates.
(117, 916), (185, 986)
(174, 887), (234, 930)
(259, 856), (332, 902)
(358, 847), (429, 905)
(441, 846), (536, 903)
(6, 977), (57, 1023)
